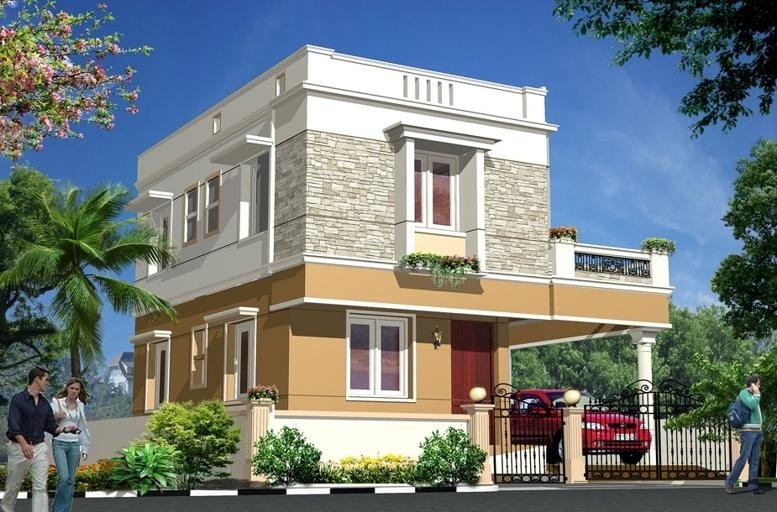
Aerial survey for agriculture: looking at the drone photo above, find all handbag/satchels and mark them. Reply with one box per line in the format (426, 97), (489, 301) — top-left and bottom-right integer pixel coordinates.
(55, 417), (63, 427)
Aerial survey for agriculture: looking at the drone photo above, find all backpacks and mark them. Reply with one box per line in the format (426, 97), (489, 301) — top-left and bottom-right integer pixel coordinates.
(727, 395), (750, 429)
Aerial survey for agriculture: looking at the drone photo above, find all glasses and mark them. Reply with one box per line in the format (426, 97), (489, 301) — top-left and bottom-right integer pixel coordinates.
(70, 428), (81, 434)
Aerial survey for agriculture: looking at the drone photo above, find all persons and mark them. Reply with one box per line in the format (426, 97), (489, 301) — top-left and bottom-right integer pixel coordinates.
(0, 367), (76, 512)
(725, 376), (764, 494)
(48, 377), (90, 511)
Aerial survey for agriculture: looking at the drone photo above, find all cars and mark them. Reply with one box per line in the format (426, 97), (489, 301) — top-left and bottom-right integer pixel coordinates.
(508, 387), (652, 465)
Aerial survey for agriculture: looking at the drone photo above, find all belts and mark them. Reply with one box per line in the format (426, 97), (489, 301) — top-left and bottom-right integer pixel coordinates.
(25, 440), (45, 445)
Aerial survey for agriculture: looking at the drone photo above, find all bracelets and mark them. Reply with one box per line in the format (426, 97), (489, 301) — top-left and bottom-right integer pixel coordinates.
(63, 427), (67, 434)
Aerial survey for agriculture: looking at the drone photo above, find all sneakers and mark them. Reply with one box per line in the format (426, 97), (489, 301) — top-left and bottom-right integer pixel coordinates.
(723, 479), (736, 494)
(749, 488), (765, 494)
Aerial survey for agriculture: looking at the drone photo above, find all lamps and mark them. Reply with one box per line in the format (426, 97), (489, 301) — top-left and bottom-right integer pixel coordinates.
(432, 326), (444, 350)
